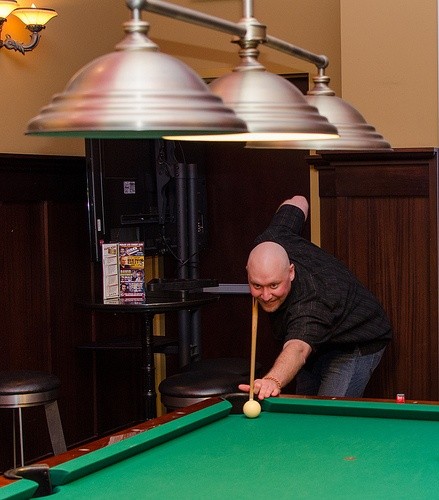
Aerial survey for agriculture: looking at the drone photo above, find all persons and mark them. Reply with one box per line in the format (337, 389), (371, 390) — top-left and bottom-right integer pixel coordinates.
(120, 283), (132, 293)
(238, 195), (392, 398)
(119, 247), (129, 256)
(132, 270), (144, 281)
(120, 256), (131, 270)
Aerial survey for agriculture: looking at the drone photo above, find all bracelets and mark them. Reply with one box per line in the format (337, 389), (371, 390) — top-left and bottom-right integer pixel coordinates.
(263, 377), (281, 389)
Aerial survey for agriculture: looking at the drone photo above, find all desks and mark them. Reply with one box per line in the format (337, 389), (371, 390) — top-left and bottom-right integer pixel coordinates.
(70, 287), (220, 425)
(0, 392), (439, 499)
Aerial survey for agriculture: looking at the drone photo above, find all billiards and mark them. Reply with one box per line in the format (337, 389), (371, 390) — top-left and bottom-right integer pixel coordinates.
(242, 400), (262, 420)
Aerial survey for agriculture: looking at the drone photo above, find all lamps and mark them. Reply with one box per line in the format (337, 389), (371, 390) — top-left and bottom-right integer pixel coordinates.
(23, 0), (397, 154)
(0, 0), (58, 55)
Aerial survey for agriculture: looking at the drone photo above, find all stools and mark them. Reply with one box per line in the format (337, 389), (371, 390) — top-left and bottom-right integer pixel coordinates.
(0, 372), (67, 465)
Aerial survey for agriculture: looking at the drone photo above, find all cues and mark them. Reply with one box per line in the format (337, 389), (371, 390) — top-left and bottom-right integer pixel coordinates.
(249, 294), (258, 403)
